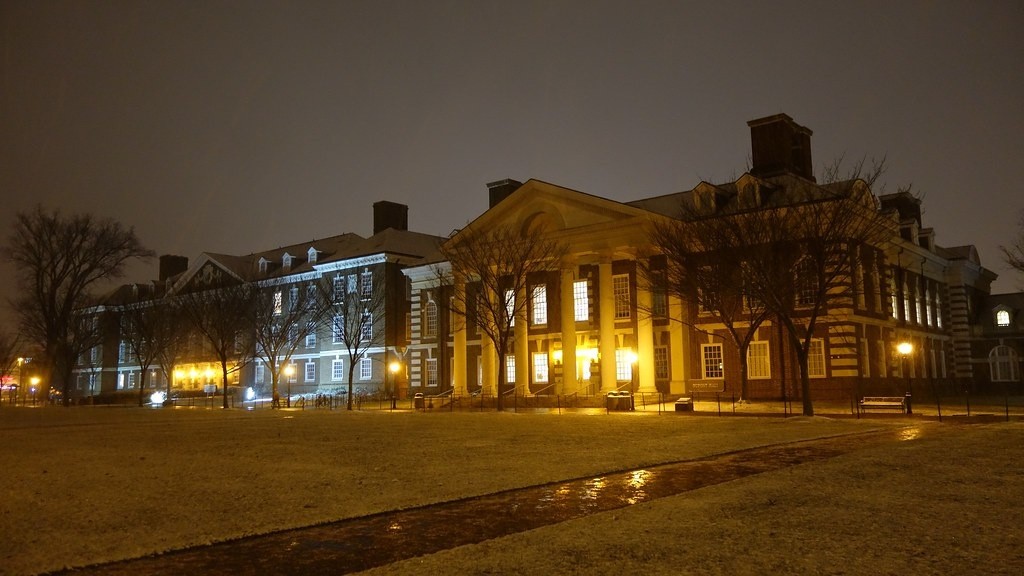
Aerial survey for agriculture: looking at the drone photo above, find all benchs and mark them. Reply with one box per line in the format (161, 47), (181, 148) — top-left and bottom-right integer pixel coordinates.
(861, 397), (905, 414)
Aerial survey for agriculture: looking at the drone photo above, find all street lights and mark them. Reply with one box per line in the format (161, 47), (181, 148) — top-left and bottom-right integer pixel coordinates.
(390, 361), (401, 409)
(626, 351), (638, 411)
(31, 377), (38, 405)
(285, 366), (295, 407)
(898, 341), (914, 413)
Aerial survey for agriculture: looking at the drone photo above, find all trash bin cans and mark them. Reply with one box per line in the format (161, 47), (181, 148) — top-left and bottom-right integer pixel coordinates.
(413, 393), (425, 409)
(674, 397), (692, 411)
(606, 391), (633, 411)
